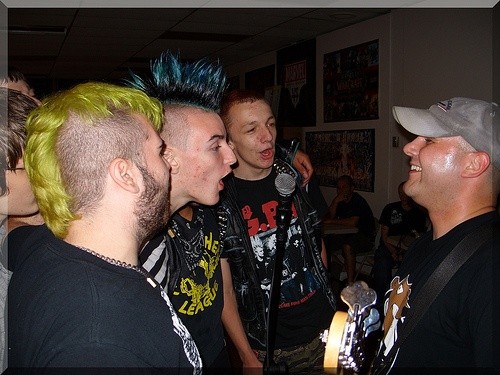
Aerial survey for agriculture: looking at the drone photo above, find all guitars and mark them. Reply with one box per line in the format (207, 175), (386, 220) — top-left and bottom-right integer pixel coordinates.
(322, 310), (356, 374)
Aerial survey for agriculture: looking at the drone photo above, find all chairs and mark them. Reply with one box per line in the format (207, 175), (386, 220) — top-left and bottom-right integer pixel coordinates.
(354, 217), (379, 280)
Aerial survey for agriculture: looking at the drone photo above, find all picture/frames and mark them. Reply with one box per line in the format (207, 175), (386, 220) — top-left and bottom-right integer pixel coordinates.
(305, 129), (375, 193)
(322, 38), (380, 123)
(244, 63), (277, 98)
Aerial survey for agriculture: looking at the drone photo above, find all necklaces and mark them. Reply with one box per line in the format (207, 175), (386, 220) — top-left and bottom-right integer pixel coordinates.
(75, 247), (148, 276)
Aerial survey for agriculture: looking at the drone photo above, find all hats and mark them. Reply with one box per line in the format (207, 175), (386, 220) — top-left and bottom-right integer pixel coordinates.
(392, 97), (500, 168)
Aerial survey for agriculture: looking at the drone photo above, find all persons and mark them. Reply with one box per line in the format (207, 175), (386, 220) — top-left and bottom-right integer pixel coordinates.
(130, 59), (313, 375)
(8, 81), (204, 375)
(367, 181), (429, 308)
(220, 95), (336, 375)
(359, 96), (500, 375)
(0, 79), (44, 375)
(320, 175), (376, 282)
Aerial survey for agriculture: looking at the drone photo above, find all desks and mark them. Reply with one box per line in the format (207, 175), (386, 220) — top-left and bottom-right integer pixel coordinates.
(323, 224), (358, 233)
(387, 235), (408, 250)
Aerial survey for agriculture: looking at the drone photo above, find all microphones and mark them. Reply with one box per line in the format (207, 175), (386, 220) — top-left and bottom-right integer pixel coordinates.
(275, 173), (296, 250)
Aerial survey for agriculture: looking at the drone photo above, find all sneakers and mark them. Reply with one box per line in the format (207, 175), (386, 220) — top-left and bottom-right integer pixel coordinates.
(339, 282), (349, 288)
